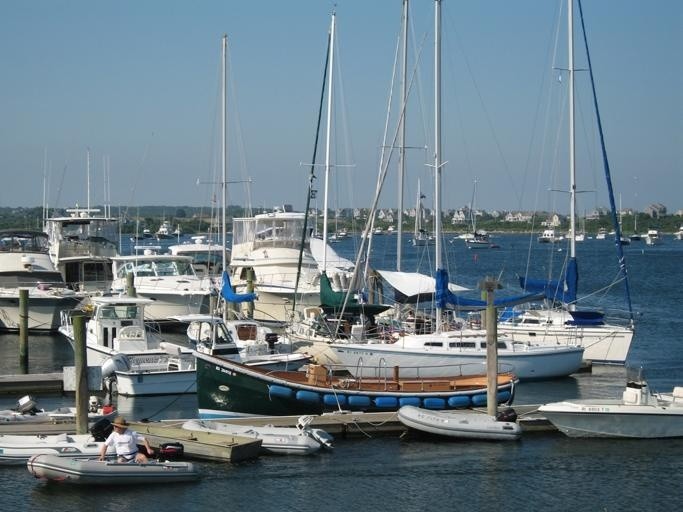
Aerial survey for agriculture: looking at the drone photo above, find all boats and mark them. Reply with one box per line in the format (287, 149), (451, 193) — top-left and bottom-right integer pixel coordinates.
(646, 230), (661, 245)
(631, 232), (640, 240)
(673, 228), (683, 240)
(538, 380), (683, 438)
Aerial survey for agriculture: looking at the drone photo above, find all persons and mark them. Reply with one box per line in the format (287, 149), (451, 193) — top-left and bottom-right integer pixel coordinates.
(98, 416), (154, 464)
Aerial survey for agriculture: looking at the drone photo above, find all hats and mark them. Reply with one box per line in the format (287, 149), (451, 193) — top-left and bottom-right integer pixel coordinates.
(111, 418), (131, 428)
